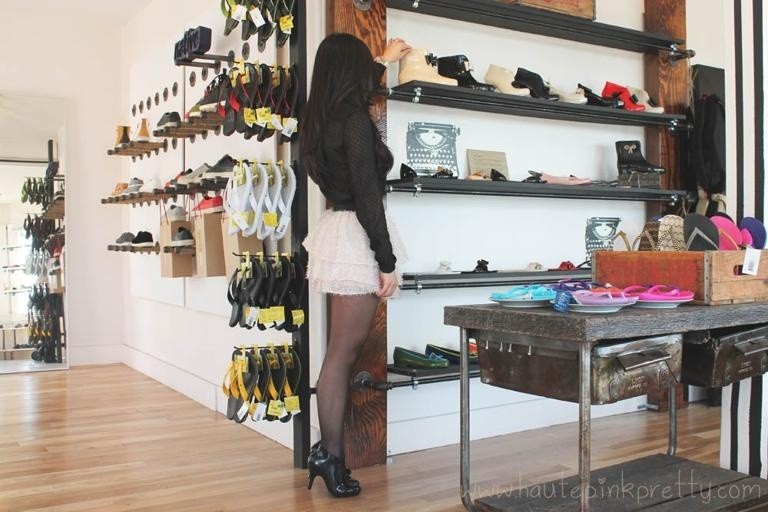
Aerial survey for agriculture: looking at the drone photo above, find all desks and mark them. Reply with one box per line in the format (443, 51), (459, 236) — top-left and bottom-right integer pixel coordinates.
(441, 301), (768, 511)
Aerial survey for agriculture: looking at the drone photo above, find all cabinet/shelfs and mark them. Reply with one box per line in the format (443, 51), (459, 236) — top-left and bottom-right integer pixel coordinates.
(382, 0), (692, 396)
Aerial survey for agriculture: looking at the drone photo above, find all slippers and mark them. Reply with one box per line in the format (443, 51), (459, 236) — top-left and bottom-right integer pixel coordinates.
(223, 348), (301, 423)
(223, 166), (297, 240)
(511, 259), (580, 272)
(424, 259), (497, 275)
(609, 213), (766, 250)
(491, 279), (696, 305)
(227, 256), (305, 332)
(217, 62), (300, 144)
(27, 283), (56, 363)
(221, 0), (295, 47)
(22, 178), (60, 275)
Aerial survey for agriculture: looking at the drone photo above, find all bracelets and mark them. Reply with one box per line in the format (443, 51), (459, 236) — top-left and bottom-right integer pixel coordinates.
(374, 56), (388, 67)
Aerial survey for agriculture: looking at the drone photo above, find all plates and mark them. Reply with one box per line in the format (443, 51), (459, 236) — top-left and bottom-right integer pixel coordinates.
(629, 297), (695, 309)
(488, 296), (555, 309)
(549, 299), (636, 314)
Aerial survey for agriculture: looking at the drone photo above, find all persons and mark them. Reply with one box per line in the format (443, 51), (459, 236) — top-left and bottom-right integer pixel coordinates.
(296, 31), (413, 505)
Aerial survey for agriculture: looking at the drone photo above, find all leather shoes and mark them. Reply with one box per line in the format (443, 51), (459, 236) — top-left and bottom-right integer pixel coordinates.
(400, 164), (618, 185)
(394, 344), (479, 368)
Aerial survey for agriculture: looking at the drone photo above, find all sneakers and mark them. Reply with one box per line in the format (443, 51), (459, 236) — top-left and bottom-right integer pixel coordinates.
(170, 227), (195, 246)
(165, 154), (237, 188)
(191, 195), (224, 216)
(157, 111), (180, 130)
(115, 231), (153, 246)
(190, 86), (226, 117)
(161, 204), (186, 221)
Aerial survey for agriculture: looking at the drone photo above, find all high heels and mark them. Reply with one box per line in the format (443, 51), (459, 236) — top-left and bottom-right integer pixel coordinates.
(307, 440), (361, 497)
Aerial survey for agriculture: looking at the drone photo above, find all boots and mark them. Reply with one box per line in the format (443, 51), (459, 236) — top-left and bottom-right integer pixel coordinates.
(397, 48), (495, 91)
(579, 83), (664, 114)
(616, 141), (665, 174)
(487, 65), (587, 104)
(110, 177), (143, 196)
(117, 119), (149, 144)
